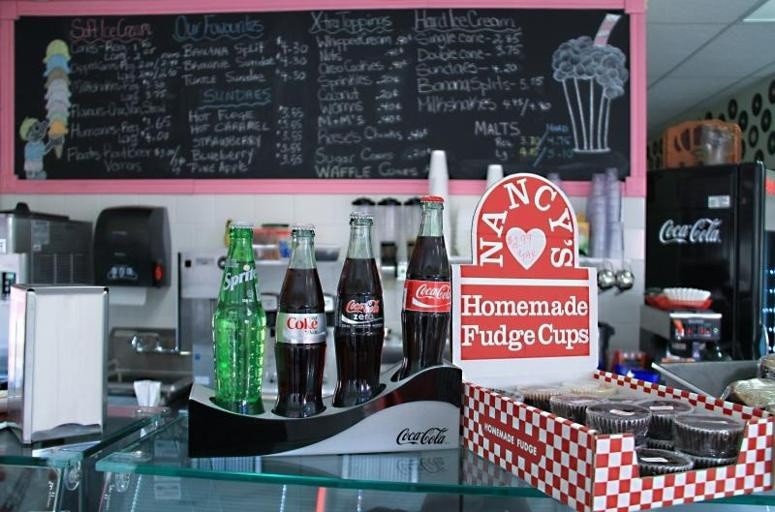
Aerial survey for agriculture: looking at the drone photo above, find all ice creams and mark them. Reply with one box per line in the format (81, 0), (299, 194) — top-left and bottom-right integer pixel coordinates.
(41, 39), (72, 159)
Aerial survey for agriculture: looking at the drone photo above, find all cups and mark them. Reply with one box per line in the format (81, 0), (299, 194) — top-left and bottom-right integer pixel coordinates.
(588, 166), (624, 261)
(430, 149), (451, 257)
(486, 164), (505, 194)
(700, 121), (732, 167)
(549, 173), (562, 186)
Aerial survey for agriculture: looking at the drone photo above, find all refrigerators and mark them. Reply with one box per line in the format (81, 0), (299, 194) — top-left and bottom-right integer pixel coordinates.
(646, 162), (774, 359)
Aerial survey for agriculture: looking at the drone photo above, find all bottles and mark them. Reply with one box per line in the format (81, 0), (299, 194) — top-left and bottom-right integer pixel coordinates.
(333, 217), (386, 406)
(400, 194), (452, 374)
(268, 230), (327, 418)
(210, 224), (269, 415)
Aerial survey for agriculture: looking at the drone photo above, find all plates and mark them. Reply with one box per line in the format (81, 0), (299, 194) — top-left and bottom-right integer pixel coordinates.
(645, 292), (712, 312)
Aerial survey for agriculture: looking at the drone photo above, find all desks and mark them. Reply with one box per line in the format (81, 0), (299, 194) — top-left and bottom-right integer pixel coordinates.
(94, 398), (775, 512)
(0, 404), (172, 512)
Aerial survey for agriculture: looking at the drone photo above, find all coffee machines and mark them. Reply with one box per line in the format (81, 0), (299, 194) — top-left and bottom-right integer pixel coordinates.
(0, 204), (96, 387)
(176, 244), (451, 396)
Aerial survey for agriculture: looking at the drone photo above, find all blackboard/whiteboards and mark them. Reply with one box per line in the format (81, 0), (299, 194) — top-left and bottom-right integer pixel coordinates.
(1, 0), (648, 198)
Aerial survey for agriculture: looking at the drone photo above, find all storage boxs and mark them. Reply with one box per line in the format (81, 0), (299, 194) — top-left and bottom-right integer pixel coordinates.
(651, 359), (762, 402)
